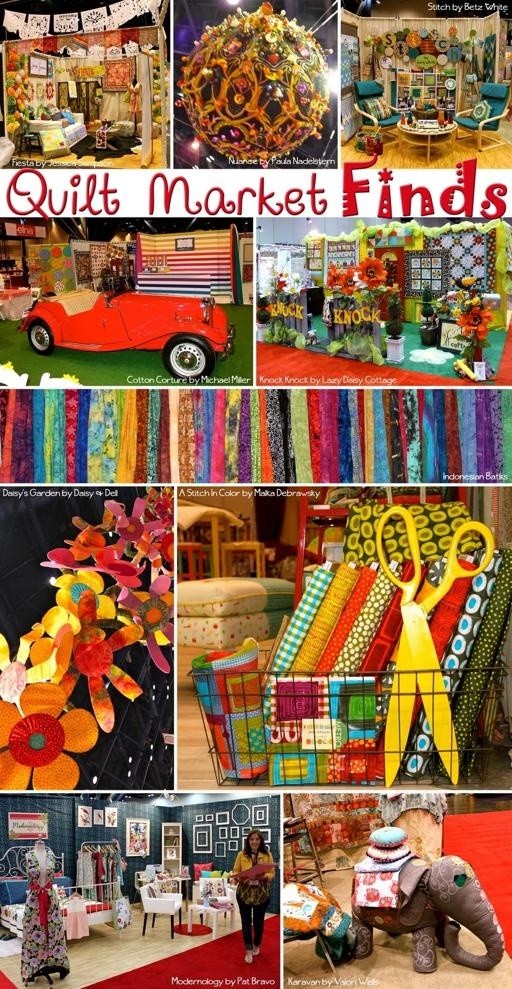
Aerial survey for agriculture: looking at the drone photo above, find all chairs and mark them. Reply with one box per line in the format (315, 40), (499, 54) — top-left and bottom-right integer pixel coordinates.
(453, 82), (512, 152)
(352, 78), (411, 139)
(133, 870), (238, 941)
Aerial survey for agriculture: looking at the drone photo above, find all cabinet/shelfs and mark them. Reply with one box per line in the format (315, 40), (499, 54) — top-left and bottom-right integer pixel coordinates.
(395, 67), (463, 113)
(161, 822), (182, 874)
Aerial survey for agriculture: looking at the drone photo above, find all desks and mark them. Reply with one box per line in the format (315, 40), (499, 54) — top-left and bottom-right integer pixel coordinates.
(16, 132), (43, 159)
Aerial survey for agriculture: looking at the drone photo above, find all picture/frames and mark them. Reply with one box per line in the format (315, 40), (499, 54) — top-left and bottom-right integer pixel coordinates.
(125, 817), (151, 857)
(7, 812), (49, 841)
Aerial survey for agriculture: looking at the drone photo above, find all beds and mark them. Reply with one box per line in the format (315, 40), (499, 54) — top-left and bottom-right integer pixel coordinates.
(0, 845), (121, 944)
(85, 125), (121, 152)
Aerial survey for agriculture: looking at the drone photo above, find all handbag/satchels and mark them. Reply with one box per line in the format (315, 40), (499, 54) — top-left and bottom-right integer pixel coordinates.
(238, 879), (270, 905)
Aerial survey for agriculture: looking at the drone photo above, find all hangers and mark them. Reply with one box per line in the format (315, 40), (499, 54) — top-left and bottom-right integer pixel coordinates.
(76, 841), (120, 857)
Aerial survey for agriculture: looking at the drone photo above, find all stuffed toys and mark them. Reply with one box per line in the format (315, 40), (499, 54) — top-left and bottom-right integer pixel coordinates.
(345, 825), (507, 976)
(282, 873), (375, 966)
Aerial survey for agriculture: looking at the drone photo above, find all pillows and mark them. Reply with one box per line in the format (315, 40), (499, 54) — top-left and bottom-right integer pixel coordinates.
(192, 861), (223, 881)
(362, 97), (392, 121)
(0, 876), (73, 905)
(470, 99), (492, 122)
(40, 103), (76, 128)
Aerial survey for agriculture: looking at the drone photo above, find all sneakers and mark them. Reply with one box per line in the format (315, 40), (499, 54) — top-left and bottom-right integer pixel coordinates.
(245, 945), (261, 963)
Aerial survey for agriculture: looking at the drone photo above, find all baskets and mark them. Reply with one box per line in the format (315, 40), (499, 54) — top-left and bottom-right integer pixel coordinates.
(186, 649), (509, 788)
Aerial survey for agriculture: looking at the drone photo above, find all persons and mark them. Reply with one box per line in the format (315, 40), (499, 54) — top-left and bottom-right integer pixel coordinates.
(227, 828), (275, 965)
(20, 837), (72, 983)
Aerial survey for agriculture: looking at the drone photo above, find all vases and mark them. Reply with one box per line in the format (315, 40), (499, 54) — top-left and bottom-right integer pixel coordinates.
(474, 346), (483, 362)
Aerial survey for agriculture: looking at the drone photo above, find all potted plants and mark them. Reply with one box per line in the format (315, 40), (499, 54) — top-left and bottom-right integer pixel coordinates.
(419, 284), (439, 346)
(384, 293), (407, 364)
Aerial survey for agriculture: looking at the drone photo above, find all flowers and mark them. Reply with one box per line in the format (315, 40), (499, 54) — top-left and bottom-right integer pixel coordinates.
(453, 274), (493, 348)
(326, 257), (389, 304)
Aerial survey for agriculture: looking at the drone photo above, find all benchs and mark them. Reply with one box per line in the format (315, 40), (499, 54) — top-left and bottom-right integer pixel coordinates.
(58, 290), (101, 316)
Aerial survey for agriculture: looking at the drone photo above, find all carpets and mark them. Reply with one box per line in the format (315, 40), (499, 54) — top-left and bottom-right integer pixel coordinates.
(70, 134), (142, 161)
(0, 936), (23, 958)
(79, 914), (280, 989)
(0, 970), (17, 989)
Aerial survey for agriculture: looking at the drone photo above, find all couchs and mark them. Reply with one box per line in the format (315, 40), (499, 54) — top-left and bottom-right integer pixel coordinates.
(27, 112), (87, 153)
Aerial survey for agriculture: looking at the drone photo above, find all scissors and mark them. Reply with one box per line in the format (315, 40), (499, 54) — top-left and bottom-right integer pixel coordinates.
(375, 507), (497, 788)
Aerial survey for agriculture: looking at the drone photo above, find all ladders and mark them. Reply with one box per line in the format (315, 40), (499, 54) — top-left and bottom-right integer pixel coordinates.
(283, 816), (325, 895)
